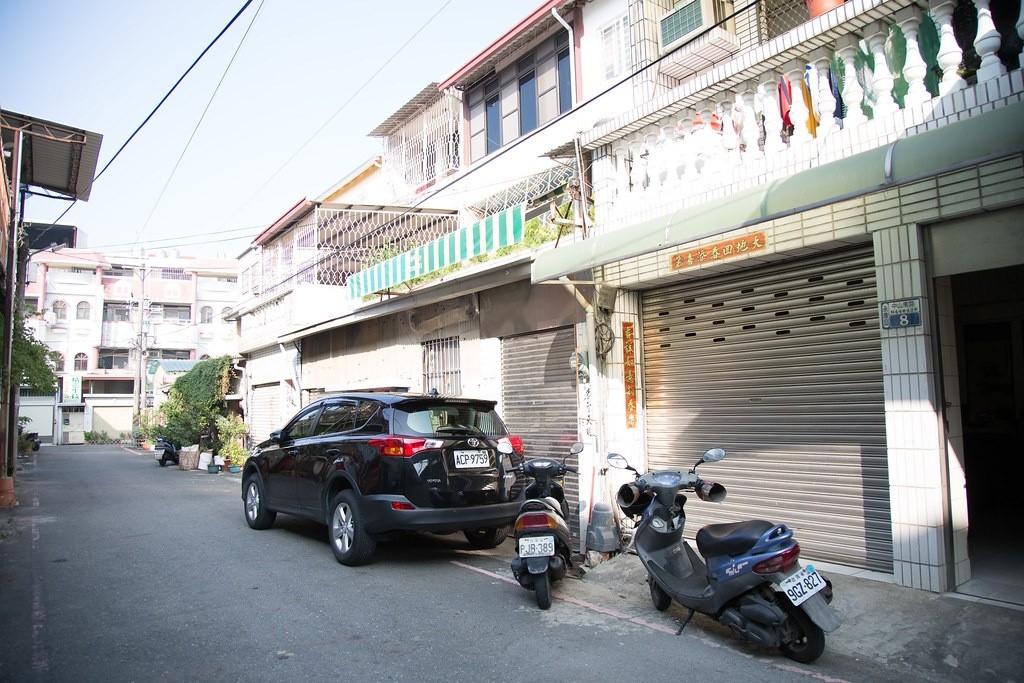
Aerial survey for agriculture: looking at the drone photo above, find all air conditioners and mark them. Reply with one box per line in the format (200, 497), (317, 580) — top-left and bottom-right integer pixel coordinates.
(656, 0), (735, 58)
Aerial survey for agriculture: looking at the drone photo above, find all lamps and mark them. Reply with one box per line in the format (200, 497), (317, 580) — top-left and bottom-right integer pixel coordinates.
(26, 242), (66, 263)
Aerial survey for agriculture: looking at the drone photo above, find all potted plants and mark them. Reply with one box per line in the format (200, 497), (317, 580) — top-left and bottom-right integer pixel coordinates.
(216, 414), (250, 472)
(21, 432), (32, 455)
(83, 431), (100, 445)
(228, 446), (242, 473)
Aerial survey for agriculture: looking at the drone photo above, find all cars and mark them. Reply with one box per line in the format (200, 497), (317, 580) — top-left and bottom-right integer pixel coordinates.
(241, 390), (528, 568)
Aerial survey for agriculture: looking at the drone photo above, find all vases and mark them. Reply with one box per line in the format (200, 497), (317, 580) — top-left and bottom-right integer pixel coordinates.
(207, 464), (220, 474)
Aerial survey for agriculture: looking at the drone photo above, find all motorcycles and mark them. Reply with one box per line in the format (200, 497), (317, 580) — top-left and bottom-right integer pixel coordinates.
(18, 425), (41, 452)
(497, 439), (587, 612)
(604, 445), (843, 665)
(153, 436), (179, 467)
